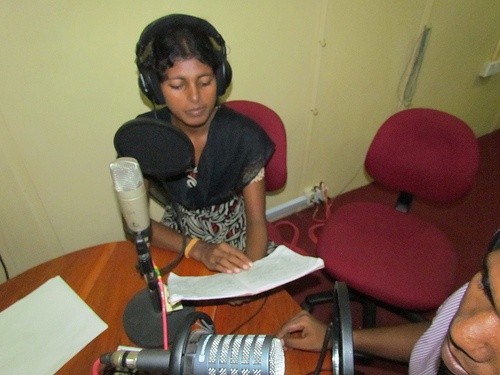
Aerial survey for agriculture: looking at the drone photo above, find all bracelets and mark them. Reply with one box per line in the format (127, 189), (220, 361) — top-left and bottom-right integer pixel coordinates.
(184, 237), (200, 259)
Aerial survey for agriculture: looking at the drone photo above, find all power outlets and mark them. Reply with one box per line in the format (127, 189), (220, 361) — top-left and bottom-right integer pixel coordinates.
(480, 60), (500, 78)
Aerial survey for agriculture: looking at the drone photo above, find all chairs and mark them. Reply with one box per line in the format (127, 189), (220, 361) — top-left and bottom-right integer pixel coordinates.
(223, 100), (287, 243)
(303, 107), (482, 363)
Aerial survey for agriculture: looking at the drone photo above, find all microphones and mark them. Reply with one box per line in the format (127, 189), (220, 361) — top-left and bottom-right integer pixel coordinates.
(113, 118), (194, 180)
(98, 333), (286, 375)
(109, 156), (151, 239)
(332, 281), (355, 375)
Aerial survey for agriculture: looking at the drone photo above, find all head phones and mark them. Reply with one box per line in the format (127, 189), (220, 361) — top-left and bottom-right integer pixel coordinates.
(136, 13), (233, 105)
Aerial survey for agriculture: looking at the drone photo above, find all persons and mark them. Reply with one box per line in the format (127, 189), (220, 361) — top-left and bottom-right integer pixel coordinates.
(277, 228), (500, 375)
(113, 14), (277, 306)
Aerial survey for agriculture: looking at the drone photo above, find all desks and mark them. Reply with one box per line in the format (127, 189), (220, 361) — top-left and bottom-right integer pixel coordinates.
(0, 242), (332, 375)
